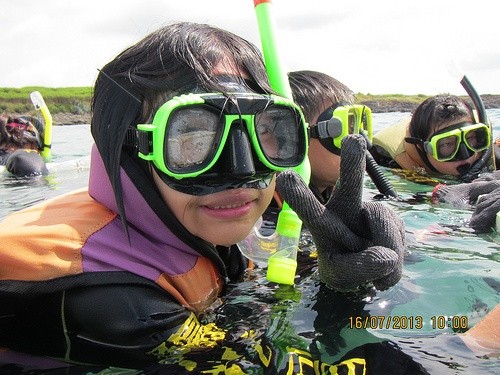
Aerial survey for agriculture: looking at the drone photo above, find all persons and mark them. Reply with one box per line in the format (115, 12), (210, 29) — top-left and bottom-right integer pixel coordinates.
(261, 71), (372, 228)
(0, 20), (500, 375)
(373, 94), (500, 183)
(0, 115), (46, 177)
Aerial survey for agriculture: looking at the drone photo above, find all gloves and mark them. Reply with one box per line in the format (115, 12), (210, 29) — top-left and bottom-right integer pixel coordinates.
(432, 172), (500, 206)
(276, 134), (405, 302)
(470, 188), (500, 230)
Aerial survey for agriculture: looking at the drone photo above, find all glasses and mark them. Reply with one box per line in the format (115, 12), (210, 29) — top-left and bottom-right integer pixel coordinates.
(308, 102), (372, 155)
(405, 121), (493, 163)
(123, 73), (309, 196)
